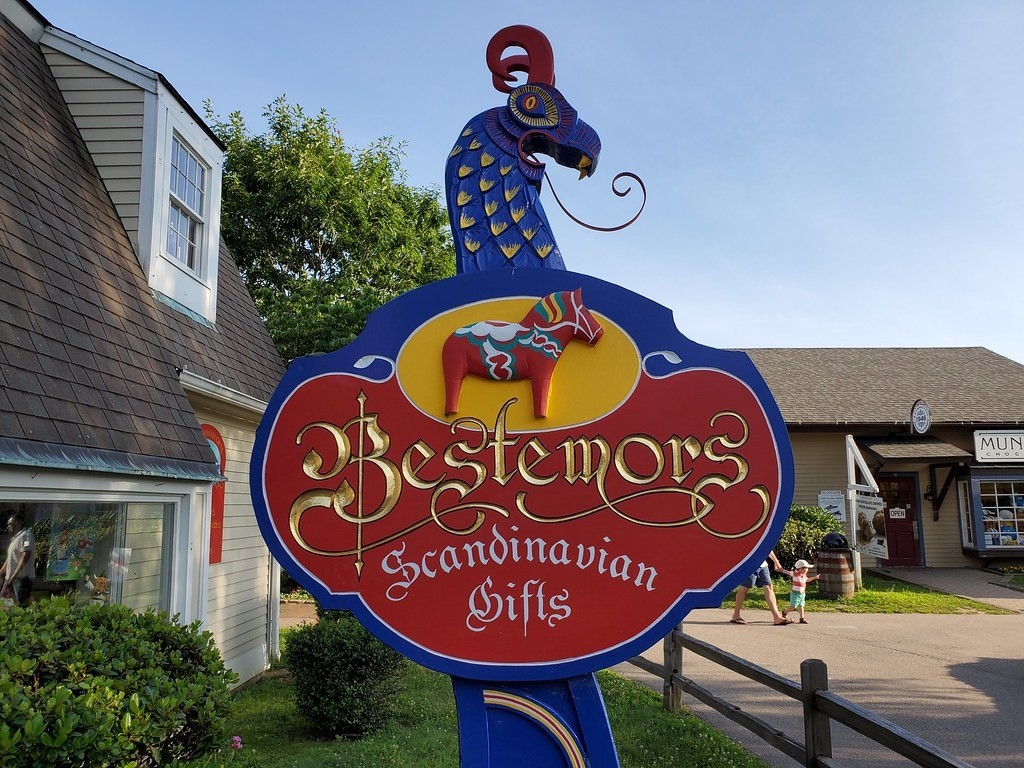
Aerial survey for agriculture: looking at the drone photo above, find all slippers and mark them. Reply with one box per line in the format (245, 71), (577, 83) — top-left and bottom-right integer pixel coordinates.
(731, 617), (746, 624)
(774, 618), (791, 625)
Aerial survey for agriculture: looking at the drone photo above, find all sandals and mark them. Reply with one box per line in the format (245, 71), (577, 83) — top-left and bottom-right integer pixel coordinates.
(782, 611), (786, 617)
(799, 618), (808, 624)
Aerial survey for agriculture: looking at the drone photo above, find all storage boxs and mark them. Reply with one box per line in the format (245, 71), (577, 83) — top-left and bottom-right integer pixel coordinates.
(984, 535), (993, 540)
(985, 539), (993, 545)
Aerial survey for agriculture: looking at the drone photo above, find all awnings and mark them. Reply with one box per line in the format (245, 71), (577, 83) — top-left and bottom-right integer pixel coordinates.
(855, 435), (973, 511)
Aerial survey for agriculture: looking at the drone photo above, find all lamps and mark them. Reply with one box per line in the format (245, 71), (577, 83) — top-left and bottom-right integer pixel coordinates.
(924, 484), (933, 501)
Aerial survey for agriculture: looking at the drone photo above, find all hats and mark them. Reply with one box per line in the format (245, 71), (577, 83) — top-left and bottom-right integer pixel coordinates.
(795, 560), (814, 569)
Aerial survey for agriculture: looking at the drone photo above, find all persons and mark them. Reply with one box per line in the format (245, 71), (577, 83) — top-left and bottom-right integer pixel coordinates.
(0, 513), (36, 608)
(775, 559), (821, 624)
(730, 551), (794, 625)
